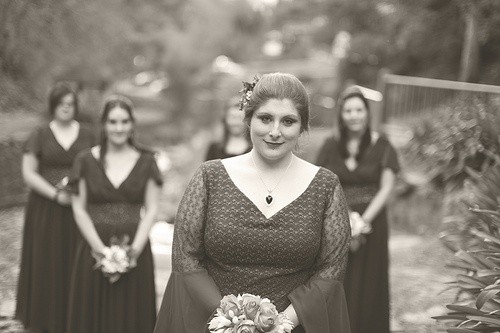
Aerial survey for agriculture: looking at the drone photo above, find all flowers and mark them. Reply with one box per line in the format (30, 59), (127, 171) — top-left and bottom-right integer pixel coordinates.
(207, 293), (295, 333)
(239, 74), (258, 111)
(89, 235), (138, 288)
(348, 210), (371, 251)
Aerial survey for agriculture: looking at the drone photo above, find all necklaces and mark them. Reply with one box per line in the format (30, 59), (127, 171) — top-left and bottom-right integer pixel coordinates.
(250, 151), (294, 207)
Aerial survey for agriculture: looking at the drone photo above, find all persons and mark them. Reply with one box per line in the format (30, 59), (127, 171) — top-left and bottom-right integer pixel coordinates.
(316, 87), (400, 333)
(204, 96), (253, 161)
(11, 82), (95, 333)
(153, 73), (352, 333)
(66, 96), (163, 333)
